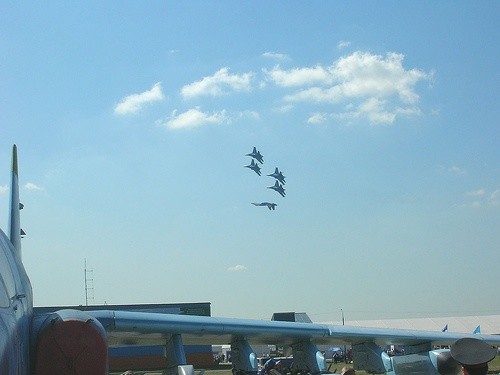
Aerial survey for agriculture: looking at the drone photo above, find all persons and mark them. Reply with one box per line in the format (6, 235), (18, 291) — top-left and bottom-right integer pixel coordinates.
(333, 352), (339, 364)
(450, 338), (498, 375)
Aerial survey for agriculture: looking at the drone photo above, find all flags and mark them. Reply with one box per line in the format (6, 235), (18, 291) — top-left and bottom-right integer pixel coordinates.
(442, 324), (447, 332)
(473, 325), (480, 333)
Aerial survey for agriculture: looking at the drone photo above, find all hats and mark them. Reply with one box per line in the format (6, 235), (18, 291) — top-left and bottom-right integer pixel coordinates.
(449, 337), (496, 368)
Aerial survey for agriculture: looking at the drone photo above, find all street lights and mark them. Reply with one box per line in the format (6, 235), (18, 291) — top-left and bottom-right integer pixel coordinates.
(341, 308), (345, 354)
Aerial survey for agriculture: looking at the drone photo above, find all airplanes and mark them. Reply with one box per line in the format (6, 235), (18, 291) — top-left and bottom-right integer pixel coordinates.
(244, 147), (286, 211)
(1, 144), (500, 375)
